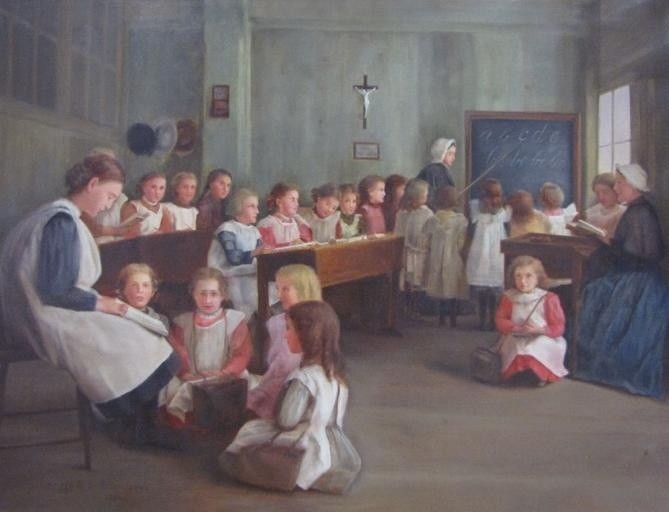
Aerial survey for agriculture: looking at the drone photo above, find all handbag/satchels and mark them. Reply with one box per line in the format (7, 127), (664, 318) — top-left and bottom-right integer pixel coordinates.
(193, 379), (247, 425)
(238, 444), (304, 492)
(468, 345), (502, 385)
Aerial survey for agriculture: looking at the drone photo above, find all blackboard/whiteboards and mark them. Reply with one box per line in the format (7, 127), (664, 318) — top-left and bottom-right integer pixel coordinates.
(464, 110), (582, 218)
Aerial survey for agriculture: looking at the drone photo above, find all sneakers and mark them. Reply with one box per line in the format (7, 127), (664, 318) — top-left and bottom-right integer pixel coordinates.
(140, 421), (186, 450)
(103, 417), (138, 446)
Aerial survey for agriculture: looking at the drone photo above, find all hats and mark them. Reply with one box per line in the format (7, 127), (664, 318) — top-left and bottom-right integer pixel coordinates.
(429, 137), (456, 163)
(616, 162), (650, 192)
